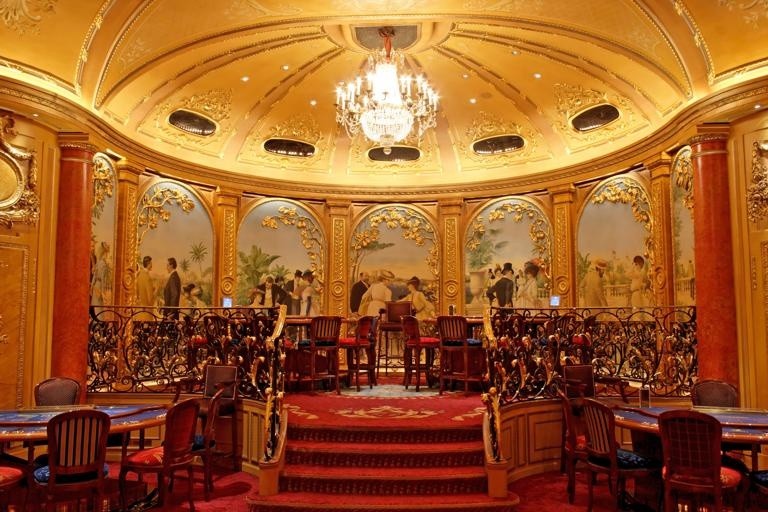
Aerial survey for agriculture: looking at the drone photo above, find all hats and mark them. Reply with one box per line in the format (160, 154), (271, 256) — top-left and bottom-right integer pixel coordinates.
(406, 276), (420, 285)
(525, 263), (539, 278)
(101, 241), (109, 253)
(247, 288), (266, 298)
(501, 263), (514, 274)
(595, 260), (607, 269)
(183, 283), (202, 296)
(293, 270), (303, 278)
(380, 270), (395, 280)
(303, 269), (314, 278)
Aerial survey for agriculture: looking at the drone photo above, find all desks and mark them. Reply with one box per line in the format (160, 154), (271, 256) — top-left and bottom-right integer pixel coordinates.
(0, 404), (171, 511)
(612, 407), (768, 511)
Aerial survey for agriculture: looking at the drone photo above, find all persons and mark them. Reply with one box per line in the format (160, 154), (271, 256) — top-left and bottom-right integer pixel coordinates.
(397, 275), (435, 320)
(160, 257), (182, 320)
(281, 269), (301, 317)
(475, 260), (542, 309)
(246, 277), (284, 315)
(577, 258), (610, 307)
(349, 269), (369, 315)
(625, 254), (655, 307)
(300, 270), (319, 318)
(358, 270), (395, 320)
(89, 240), (115, 320)
(136, 254), (156, 318)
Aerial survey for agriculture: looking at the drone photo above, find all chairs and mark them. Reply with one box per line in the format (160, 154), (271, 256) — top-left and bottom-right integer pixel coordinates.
(403, 305), (622, 398)
(691, 379), (761, 456)
(658, 409), (748, 511)
(581, 399), (658, 512)
(33, 410), (110, 512)
(553, 380), (599, 492)
(23, 378), (81, 448)
(158, 389), (224, 494)
(561, 365), (594, 469)
(0, 465), (24, 512)
(119, 398), (200, 510)
(744, 470), (768, 511)
(375, 300), (413, 379)
(184, 303), (382, 395)
(174, 364), (240, 477)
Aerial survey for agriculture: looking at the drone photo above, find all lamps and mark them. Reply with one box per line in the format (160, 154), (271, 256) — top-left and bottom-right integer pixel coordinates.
(332, 25), (441, 157)
